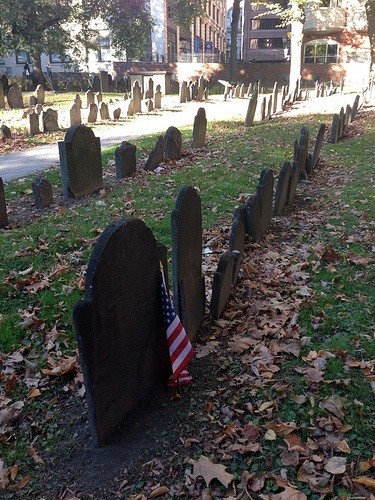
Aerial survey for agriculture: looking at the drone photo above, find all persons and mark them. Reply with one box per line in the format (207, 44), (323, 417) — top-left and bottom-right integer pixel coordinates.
(46, 62), (53, 79)
(24, 61), (30, 79)
(1, 74), (9, 96)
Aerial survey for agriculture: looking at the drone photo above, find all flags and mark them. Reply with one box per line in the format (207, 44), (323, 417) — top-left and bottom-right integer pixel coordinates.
(160, 262), (195, 387)
